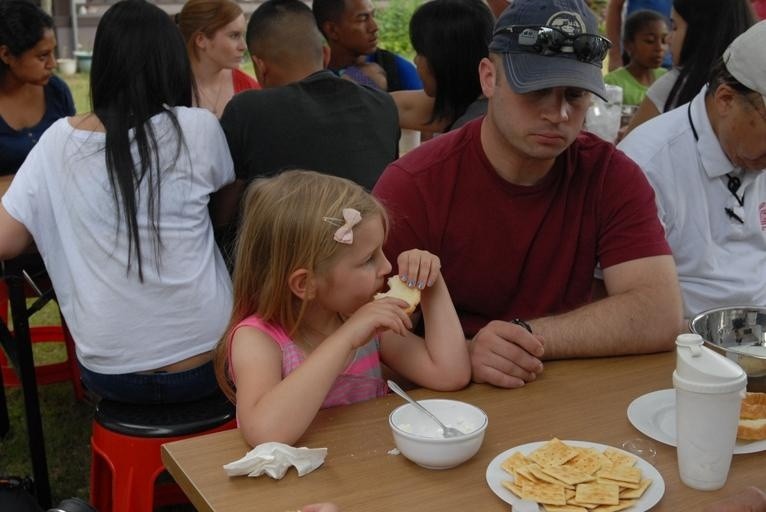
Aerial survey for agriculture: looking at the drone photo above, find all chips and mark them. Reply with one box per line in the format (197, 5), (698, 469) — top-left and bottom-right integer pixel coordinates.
(501, 437), (652, 512)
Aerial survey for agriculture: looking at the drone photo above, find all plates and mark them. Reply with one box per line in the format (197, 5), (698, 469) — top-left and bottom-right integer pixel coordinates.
(626, 386), (766, 455)
(486, 439), (666, 512)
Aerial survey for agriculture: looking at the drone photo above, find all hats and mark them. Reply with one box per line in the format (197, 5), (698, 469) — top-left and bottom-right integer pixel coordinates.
(721, 19), (766, 105)
(488, 0), (610, 105)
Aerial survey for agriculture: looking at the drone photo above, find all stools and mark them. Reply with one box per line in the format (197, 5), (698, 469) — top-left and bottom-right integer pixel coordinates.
(87, 396), (237, 512)
(1, 272), (86, 401)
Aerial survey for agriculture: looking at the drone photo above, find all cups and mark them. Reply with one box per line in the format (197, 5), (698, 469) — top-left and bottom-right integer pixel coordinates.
(56, 58), (77, 74)
(621, 440), (657, 466)
(670, 331), (749, 494)
(585, 84), (623, 144)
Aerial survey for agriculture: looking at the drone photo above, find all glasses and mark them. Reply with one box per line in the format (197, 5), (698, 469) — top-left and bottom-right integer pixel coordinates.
(492, 23), (614, 63)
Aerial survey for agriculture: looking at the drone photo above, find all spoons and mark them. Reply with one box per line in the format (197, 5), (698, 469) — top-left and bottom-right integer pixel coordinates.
(383, 379), (465, 439)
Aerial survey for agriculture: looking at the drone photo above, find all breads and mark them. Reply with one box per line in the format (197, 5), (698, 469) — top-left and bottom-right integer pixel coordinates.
(736, 393), (766, 440)
(374, 275), (423, 317)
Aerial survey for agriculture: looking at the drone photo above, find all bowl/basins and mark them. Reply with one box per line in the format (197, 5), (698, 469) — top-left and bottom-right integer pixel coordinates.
(685, 304), (766, 379)
(387, 397), (488, 472)
(620, 106), (640, 124)
(72, 52), (93, 74)
